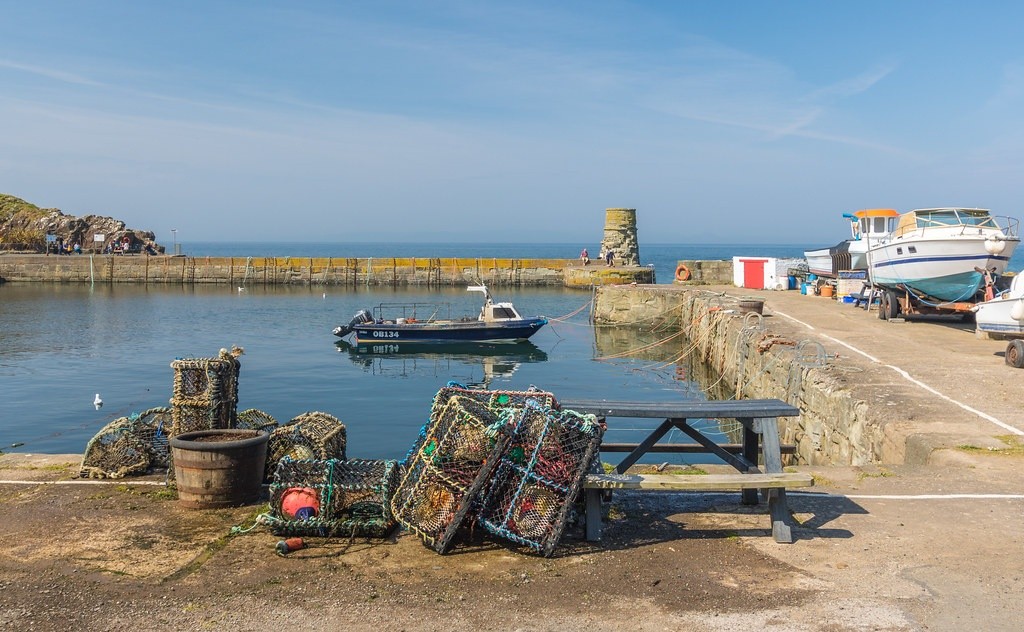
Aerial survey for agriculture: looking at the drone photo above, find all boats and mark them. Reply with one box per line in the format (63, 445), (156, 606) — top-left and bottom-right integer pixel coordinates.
(863, 205), (1022, 303)
(803, 210), (902, 279)
(968, 268), (1024, 342)
(330, 255), (549, 345)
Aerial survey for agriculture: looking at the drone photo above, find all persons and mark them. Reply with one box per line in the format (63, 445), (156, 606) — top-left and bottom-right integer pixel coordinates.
(580, 249), (589, 266)
(108, 237), (130, 255)
(600, 250), (616, 269)
(56, 236), (83, 256)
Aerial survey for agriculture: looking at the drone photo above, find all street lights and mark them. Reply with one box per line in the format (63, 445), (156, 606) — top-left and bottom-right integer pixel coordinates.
(171, 229), (178, 255)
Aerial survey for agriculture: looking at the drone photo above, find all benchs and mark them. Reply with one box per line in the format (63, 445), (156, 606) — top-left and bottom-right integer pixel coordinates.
(598, 443), (796, 454)
(850, 293), (864, 300)
(579, 473), (814, 491)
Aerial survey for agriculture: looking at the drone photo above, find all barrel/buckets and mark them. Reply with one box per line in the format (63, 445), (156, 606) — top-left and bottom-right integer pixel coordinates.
(396, 317), (406, 324)
(169, 429), (271, 508)
(771, 273), (833, 297)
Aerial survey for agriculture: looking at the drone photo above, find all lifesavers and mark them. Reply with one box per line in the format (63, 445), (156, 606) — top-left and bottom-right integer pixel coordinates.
(123, 237), (130, 245)
(675, 264), (691, 282)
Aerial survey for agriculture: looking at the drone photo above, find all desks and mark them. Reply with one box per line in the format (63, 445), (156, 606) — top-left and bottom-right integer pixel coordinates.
(559, 399), (800, 543)
(855, 282), (873, 310)
